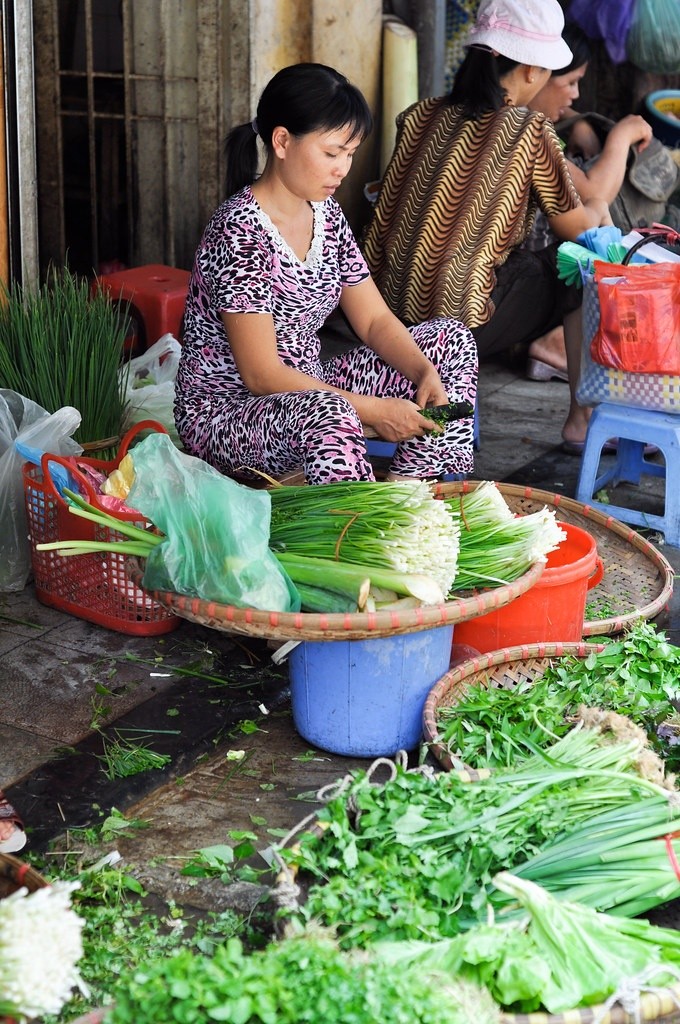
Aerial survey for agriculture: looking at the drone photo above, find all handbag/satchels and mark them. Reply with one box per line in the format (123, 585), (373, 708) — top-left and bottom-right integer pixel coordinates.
(573, 125), (679, 236)
(123, 432), (303, 615)
(574, 229), (680, 415)
(117, 332), (189, 449)
(0, 388), (87, 594)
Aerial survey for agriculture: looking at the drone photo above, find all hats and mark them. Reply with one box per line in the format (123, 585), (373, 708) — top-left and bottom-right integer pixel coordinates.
(460, 0), (575, 71)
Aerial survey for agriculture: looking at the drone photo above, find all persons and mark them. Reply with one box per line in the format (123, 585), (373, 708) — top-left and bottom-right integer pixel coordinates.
(360, 0), (617, 450)
(511, 14), (655, 382)
(158, 58), (485, 488)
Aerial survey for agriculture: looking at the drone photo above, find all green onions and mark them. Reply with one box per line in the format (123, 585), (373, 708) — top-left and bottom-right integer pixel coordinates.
(0, 267), (563, 614)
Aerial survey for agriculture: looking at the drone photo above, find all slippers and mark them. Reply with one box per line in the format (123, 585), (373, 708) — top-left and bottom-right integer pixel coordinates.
(562, 437), (659, 456)
(526, 358), (569, 383)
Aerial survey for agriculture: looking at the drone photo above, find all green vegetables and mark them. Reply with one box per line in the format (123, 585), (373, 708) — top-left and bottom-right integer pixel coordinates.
(0, 619), (680, 1023)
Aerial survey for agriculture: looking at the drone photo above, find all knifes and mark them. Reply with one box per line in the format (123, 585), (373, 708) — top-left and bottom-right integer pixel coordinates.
(416, 402), (476, 424)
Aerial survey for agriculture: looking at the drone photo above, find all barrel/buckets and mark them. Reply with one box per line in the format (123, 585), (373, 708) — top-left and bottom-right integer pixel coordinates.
(448, 519), (608, 670)
(269, 618), (460, 761)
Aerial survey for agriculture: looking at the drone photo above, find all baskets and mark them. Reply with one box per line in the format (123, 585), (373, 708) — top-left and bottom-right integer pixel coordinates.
(421, 643), (680, 773)
(20, 420), (181, 636)
(430, 479), (676, 635)
(124, 546), (547, 643)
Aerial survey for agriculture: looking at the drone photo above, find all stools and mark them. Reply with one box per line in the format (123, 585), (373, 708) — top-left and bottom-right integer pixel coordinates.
(93, 264), (192, 366)
(574, 403), (679, 547)
